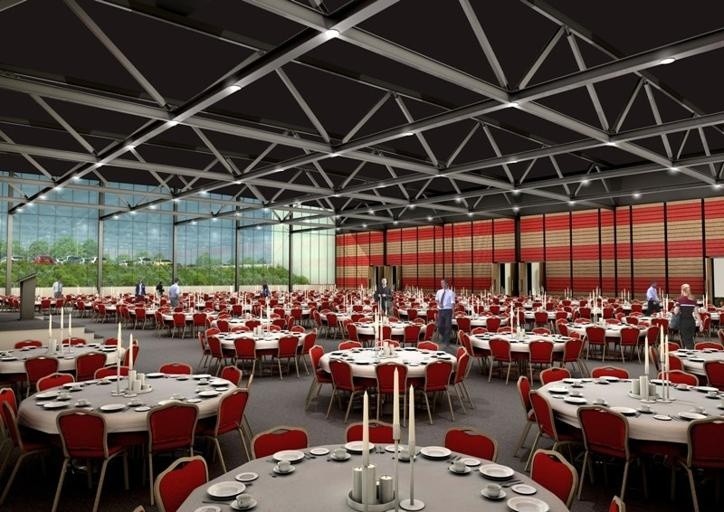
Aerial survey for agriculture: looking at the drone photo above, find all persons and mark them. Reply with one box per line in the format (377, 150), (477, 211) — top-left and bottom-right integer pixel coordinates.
(135, 278), (146, 296)
(646, 281), (658, 313)
(373, 277), (392, 315)
(263, 281), (270, 297)
(156, 280), (165, 298)
(170, 278), (181, 308)
(52, 278), (62, 296)
(674, 284), (698, 349)
(435, 278), (456, 347)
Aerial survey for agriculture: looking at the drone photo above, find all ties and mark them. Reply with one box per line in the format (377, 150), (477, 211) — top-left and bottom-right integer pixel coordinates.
(440, 291), (445, 308)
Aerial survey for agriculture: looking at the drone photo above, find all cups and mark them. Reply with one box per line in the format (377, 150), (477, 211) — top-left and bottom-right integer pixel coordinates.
(453, 459), (465, 472)
(236, 494), (251, 508)
(398, 446), (410, 460)
(334, 446), (347, 458)
(486, 483), (500, 496)
(277, 459), (291, 473)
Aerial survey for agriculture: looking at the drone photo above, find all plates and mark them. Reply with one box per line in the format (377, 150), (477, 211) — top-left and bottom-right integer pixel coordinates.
(383, 444), (402, 453)
(310, 447), (330, 455)
(448, 465), (472, 474)
(481, 487), (505, 499)
(206, 480), (246, 500)
(397, 453), (415, 462)
(460, 457), (481, 466)
(330, 452), (351, 461)
(192, 506), (221, 512)
(477, 463), (515, 478)
(511, 484), (537, 495)
(477, 332), (573, 344)
(273, 450), (305, 463)
(419, 446), (451, 458)
(1, 342), (118, 361)
(674, 346), (719, 363)
(566, 317), (654, 332)
(36, 371), (230, 413)
(272, 464), (296, 474)
(550, 377), (724, 422)
(210, 316), (303, 342)
(333, 347), (452, 365)
(354, 320), (423, 330)
(229, 499), (256, 510)
(234, 472), (259, 480)
(505, 496), (550, 511)
(344, 440), (375, 452)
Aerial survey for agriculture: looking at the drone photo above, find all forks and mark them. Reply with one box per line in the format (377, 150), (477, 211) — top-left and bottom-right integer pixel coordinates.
(498, 478), (524, 488)
(303, 452), (315, 461)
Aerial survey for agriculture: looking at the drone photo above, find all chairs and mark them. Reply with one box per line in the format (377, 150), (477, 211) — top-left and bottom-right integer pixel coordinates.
(0, 288), (723, 512)
